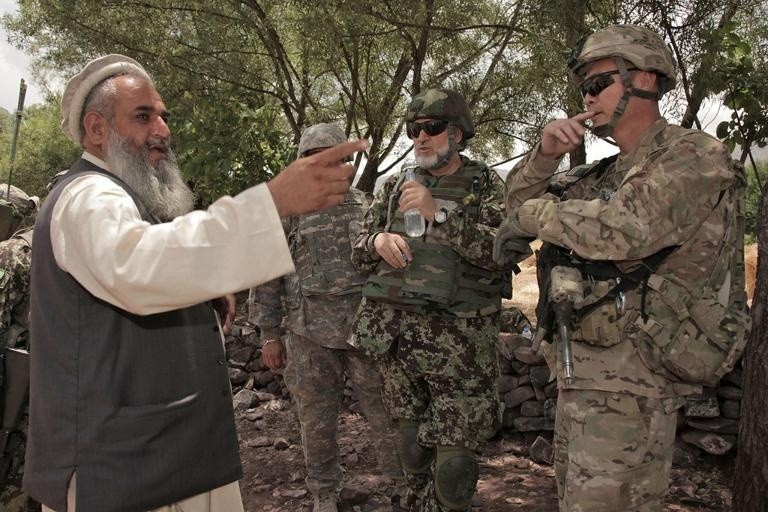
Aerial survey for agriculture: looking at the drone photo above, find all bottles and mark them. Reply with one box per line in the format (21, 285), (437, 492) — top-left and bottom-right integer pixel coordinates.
(522, 327), (532, 337)
(402, 167), (427, 239)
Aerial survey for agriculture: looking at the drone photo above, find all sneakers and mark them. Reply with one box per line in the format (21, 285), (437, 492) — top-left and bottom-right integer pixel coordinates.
(312, 490), (340, 512)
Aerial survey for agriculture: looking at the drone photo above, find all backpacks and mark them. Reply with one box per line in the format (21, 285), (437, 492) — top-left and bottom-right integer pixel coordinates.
(664, 297), (753, 387)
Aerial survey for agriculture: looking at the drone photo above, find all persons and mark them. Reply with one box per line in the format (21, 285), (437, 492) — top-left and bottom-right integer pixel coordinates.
(22, 52), (372, 511)
(244, 121), (372, 511)
(346, 84), (520, 511)
(489, 24), (737, 512)
(0, 225), (36, 510)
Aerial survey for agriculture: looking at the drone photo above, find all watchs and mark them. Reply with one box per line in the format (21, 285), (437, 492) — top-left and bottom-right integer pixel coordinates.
(428, 206), (450, 230)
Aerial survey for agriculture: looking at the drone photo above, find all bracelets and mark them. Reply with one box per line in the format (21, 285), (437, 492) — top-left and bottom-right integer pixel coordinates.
(259, 337), (279, 347)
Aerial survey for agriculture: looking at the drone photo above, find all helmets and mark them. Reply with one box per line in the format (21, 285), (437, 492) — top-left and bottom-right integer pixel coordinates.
(570, 26), (678, 95)
(405, 88), (477, 140)
(296, 123), (355, 161)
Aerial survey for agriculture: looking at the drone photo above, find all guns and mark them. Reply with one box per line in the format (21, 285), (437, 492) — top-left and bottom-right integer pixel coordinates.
(531, 241), (584, 387)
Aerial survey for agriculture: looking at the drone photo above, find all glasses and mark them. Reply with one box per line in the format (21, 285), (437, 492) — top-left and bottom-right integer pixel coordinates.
(407, 118), (448, 139)
(580, 66), (638, 99)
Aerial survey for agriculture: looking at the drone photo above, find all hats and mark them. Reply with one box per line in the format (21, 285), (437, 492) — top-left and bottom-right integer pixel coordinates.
(60, 54), (146, 148)
